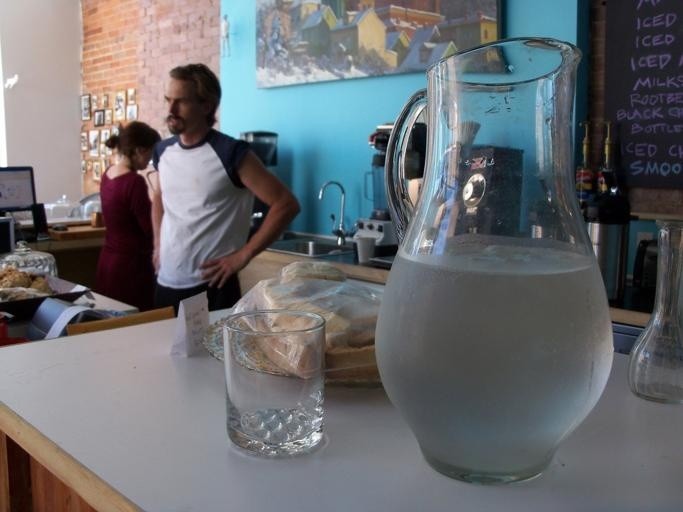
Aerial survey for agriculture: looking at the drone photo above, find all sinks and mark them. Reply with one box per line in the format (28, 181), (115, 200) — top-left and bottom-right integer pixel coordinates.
(271, 231), (311, 243)
(266, 233), (354, 257)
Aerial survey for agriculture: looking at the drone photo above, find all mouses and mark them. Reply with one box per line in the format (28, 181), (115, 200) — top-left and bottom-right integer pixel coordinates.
(53, 224), (68, 231)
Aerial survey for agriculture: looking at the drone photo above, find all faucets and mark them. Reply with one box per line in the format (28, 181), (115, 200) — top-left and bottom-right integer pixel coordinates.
(318, 181), (345, 247)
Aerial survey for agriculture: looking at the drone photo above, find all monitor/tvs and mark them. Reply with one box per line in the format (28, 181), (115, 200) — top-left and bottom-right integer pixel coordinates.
(0, 217), (14, 254)
(0, 167), (36, 218)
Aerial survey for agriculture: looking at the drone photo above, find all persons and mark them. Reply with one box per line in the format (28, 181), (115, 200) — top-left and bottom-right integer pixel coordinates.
(94, 119), (162, 312)
(147, 61), (301, 315)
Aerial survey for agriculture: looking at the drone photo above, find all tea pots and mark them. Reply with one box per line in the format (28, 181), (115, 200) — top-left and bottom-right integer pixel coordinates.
(361, 152), (394, 223)
(374, 36), (614, 487)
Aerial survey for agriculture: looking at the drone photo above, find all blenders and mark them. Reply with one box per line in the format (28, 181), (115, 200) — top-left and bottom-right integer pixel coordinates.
(350, 119), (429, 258)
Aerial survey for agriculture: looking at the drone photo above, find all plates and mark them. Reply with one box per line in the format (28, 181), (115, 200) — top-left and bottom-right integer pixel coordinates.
(0, 267), (91, 323)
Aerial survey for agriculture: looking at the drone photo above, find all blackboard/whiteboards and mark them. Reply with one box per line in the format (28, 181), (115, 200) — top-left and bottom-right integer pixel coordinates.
(605, 0), (683, 189)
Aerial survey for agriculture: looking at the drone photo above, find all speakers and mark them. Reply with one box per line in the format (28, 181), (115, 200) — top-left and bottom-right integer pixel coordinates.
(32, 203), (47, 235)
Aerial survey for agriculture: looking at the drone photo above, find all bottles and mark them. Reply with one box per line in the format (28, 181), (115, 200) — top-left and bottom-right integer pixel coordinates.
(580, 194), (630, 301)
(626, 225), (682, 403)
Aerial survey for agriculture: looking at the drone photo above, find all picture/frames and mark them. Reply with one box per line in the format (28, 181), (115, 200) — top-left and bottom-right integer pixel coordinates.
(78, 88), (138, 184)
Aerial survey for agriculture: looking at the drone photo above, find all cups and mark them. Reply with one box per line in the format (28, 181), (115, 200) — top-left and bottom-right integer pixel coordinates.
(222, 308), (326, 458)
(355, 236), (377, 265)
